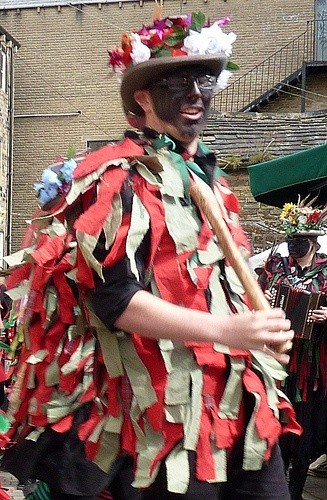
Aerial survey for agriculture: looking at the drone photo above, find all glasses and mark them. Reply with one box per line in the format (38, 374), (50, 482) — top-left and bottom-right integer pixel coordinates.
(285, 237), (310, 244)
(148, 75), (217, 89)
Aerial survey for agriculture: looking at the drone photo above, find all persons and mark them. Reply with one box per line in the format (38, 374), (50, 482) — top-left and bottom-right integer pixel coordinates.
(258, 202), (327, 500)
(9, 14), (294, 500)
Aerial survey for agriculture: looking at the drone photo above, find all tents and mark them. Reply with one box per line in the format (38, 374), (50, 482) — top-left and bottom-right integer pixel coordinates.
(246, 145), (326, 198)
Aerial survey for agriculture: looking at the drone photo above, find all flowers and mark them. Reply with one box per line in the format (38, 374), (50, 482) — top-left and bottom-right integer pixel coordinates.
(110, 12), (241, 94)
(280, 194), (327, 238)
(33, 145), (79, 208)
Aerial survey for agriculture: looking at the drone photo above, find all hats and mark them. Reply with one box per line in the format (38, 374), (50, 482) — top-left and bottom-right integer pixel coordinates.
(279, 193), (327, 236)
(106, 12), (241, 129)
